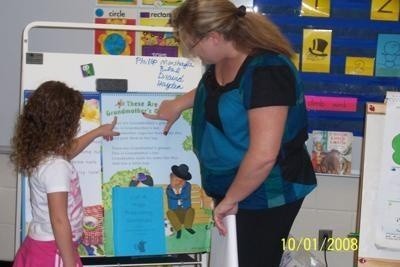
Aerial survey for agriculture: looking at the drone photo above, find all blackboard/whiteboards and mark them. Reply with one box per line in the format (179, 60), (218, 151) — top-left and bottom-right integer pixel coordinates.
(18, 52), (212, 258)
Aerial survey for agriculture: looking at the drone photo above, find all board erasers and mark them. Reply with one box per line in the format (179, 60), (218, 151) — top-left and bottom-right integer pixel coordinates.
(96, 79), (128, 92)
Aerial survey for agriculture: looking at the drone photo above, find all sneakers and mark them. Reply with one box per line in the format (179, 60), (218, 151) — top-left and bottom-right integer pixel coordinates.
(176, 229), (182, 239)
(185, 228), (196, 234)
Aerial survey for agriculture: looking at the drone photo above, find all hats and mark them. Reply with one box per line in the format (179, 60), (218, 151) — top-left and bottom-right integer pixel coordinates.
(308, 38), (329, 56)
(171, 164), (192, 180)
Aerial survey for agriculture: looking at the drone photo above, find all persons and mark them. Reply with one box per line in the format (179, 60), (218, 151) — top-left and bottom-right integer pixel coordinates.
(8, 80), (121, 266)
(141, 0), (318, 265)
